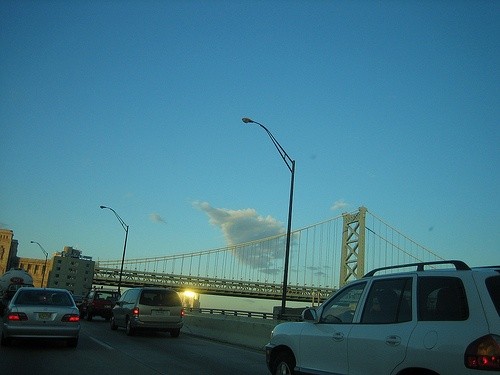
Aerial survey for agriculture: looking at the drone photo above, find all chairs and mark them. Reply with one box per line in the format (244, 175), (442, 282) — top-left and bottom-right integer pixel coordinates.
(51, 295), (64, 304)
(22, 292), (34, 301)
(153, 296), (159, 301)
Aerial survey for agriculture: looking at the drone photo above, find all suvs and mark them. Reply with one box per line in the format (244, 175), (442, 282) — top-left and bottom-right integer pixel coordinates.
(267, 260), (500, 375)
(81, 287), (119, 320)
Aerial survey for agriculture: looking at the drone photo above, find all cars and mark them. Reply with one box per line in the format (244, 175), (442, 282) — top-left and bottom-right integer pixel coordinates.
(73, 294), (93, 313)
(3, 287), (80, 346)
(111, 287), (184, 338)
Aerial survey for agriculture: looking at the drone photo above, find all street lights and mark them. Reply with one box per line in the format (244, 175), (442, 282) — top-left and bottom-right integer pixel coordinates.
(100, 205), (129, 286)
(242, 116), (296, 314)
(30, 240), (49, 288)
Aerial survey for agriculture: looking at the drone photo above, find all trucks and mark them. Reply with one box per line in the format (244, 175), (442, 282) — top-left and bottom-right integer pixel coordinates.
(0, 268), (34, 316)
